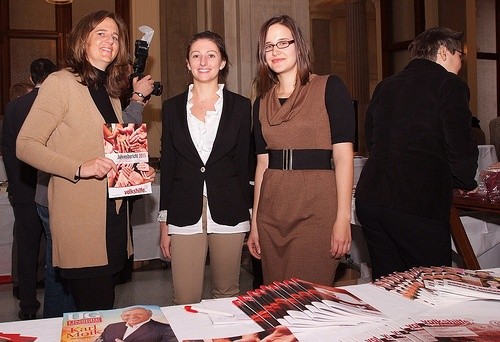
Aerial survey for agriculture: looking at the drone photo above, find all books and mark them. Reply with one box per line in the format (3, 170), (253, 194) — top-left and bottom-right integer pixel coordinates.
(0, 332), (37, 342)
(370, 266), (500, 307)
(103, 122), (153, 198)
(335, 317), (500, 342)
(182, 327), (301, 342)
(61, 304), (178, 342)
(203, 277), (382, 331)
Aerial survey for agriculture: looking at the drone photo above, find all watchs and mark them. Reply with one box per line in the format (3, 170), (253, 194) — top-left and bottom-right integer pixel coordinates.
(132, 91), (146, 98)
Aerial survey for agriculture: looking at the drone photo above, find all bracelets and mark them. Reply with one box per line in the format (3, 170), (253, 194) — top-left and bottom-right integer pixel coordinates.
(130, 98), (145, 106)
(79, 165), (81, 178)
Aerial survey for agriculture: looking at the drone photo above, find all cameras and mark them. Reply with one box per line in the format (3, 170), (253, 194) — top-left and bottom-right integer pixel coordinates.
(127, 39), (163, 97)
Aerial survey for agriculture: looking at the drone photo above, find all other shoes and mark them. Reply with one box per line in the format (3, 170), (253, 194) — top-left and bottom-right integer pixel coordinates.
(18, 300), (37, 321)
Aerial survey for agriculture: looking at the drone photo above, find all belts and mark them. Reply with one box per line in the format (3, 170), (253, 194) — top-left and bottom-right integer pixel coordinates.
(267, 148), (333, 170)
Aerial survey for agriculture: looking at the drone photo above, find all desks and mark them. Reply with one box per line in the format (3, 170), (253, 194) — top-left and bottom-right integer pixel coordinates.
(0, 144), (500, 282)
(449, 194), (500, 270)
(0, 268), (500, 342)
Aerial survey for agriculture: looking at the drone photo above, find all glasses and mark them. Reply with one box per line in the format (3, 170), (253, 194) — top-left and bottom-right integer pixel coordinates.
(264, 39), (295, 52)
(447, 45), (466, 60)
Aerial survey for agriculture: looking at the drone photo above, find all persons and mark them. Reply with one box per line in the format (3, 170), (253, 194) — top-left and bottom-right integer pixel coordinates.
(471, 116), (486, 146)
(93, 307), (179, 342)
(2, 59), (58, 320)
(489, 117), (500, 162)
(249, 126), (263, 291)
(34, 58), (155, 316)
(15, 10), (156, 318)
(157, 32), (252, 305)
(353, 28), (478, 282)
(247, 15), (354, 288)
